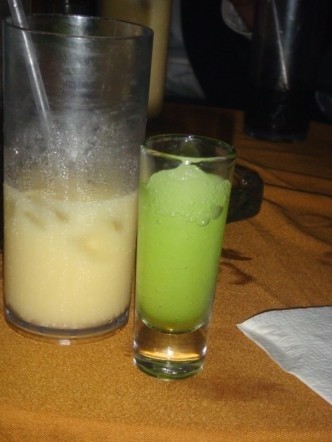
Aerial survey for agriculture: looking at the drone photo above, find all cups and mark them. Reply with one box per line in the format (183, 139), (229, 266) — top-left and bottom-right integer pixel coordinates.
(132, 130), (239, 380)
(2, 10), (154, 345)
(243, 1), (321, 143)
